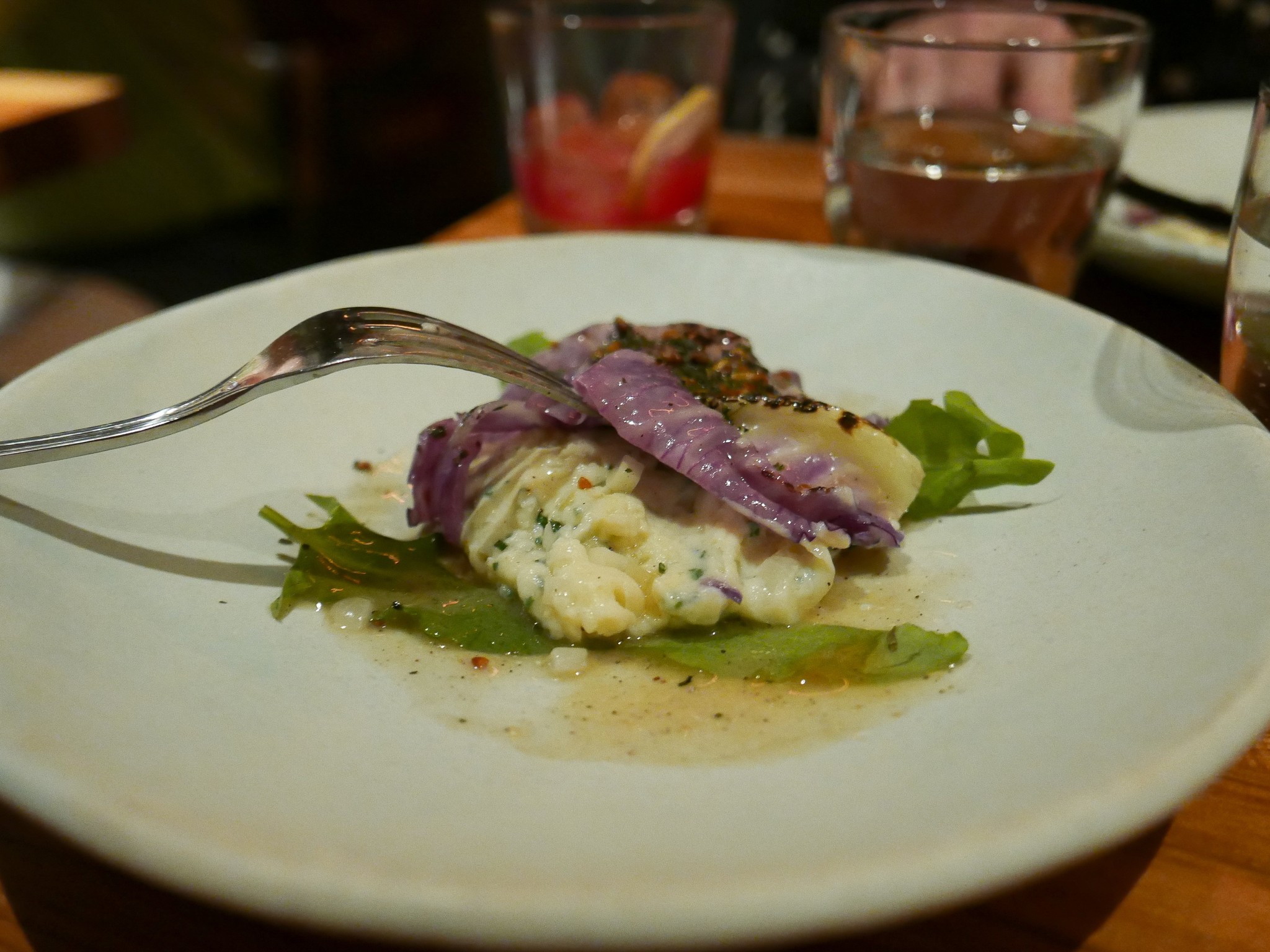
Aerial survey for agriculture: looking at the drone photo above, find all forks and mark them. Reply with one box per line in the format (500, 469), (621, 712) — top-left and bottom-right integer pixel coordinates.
(0, 305), (611, 472)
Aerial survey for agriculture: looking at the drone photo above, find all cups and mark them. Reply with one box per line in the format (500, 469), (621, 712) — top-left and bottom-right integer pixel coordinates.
(817, 2), (1155, 296)
(488, 1), (740, 238)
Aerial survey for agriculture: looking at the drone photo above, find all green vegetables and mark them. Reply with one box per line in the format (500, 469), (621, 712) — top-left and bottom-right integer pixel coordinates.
(884, 390), (1055, 519)
(258, 494), (970, 687)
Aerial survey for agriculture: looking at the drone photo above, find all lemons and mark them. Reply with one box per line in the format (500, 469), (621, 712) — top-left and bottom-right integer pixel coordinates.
(626, 84), (716, 190)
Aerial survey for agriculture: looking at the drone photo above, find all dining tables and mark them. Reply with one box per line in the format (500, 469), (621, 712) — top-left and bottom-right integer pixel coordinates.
(0, 112), (1269, 952)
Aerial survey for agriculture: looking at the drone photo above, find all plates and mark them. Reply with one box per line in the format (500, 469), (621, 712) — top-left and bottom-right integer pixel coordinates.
(3, 234), (1270, 951)
